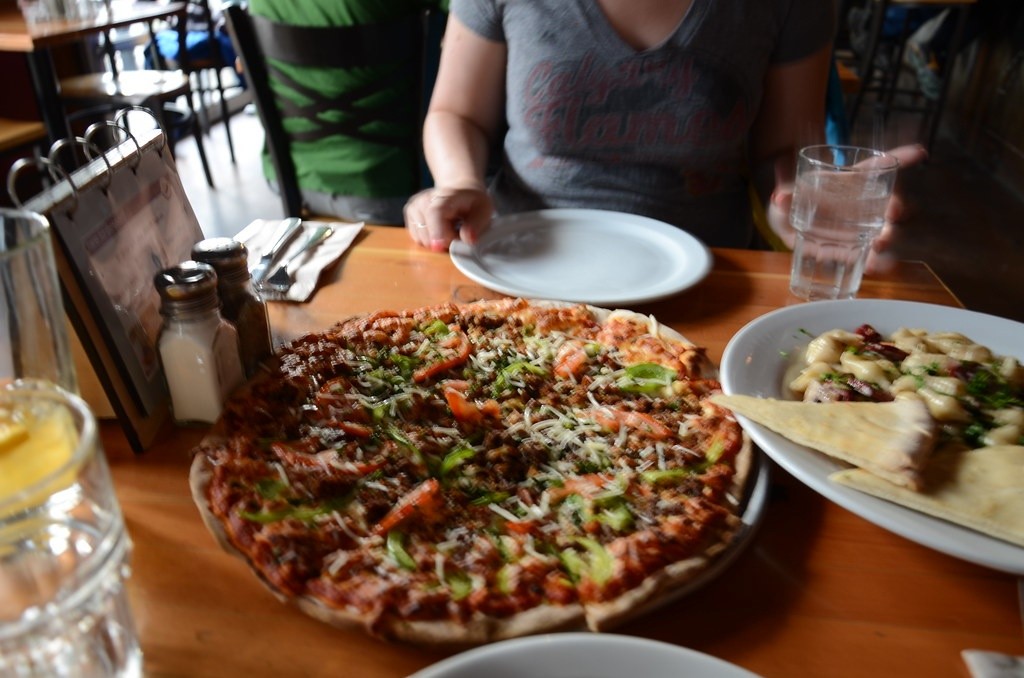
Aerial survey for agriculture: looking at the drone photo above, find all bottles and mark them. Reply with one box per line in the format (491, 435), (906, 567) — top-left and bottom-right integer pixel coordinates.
(153, 237), (275, 429)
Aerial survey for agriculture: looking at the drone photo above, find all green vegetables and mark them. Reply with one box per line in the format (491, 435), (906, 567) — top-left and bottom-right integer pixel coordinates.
(778, 329), (1024, 448)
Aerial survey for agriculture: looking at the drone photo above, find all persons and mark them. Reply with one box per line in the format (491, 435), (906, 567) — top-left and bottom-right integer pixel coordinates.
(235, 0), (449, 228)
(402, 0), (926, 280)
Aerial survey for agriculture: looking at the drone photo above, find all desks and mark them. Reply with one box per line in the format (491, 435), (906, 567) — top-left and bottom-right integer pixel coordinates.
(94, 219), (1024, 678)
(0, 3), (188, 174)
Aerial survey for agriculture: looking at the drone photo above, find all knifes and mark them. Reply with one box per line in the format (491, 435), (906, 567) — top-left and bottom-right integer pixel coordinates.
(250, 217), (302, 282)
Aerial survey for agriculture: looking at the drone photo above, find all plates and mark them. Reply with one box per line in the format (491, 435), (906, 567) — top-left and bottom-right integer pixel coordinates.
(448, 207), (714, 307)
(719, 298), (1024, 574)
(407, 631), (765, 678)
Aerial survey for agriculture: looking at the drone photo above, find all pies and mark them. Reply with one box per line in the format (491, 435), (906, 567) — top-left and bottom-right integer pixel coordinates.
(828, 442), (1024, 547)
(705, 393), (938, 493)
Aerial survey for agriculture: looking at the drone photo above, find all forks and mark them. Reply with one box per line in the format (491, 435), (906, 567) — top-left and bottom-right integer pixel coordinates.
(253, 225), (336, 295)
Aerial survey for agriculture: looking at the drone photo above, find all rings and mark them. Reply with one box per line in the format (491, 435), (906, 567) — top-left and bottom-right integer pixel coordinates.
(416, 224), (426, 228)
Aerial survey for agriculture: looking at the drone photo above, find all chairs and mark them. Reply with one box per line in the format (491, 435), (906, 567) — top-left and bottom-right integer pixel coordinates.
(220, 5), (446, 220)
(60, 0), (219, 192)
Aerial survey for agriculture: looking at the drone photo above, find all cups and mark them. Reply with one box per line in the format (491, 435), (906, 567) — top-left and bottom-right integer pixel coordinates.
(786, 143), (902, 301)
(0, 209), (81, 399)
(1, 375), (146, 677)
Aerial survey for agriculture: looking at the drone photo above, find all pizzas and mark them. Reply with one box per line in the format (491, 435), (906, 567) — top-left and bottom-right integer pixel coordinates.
(186, 296), (756, 646)
